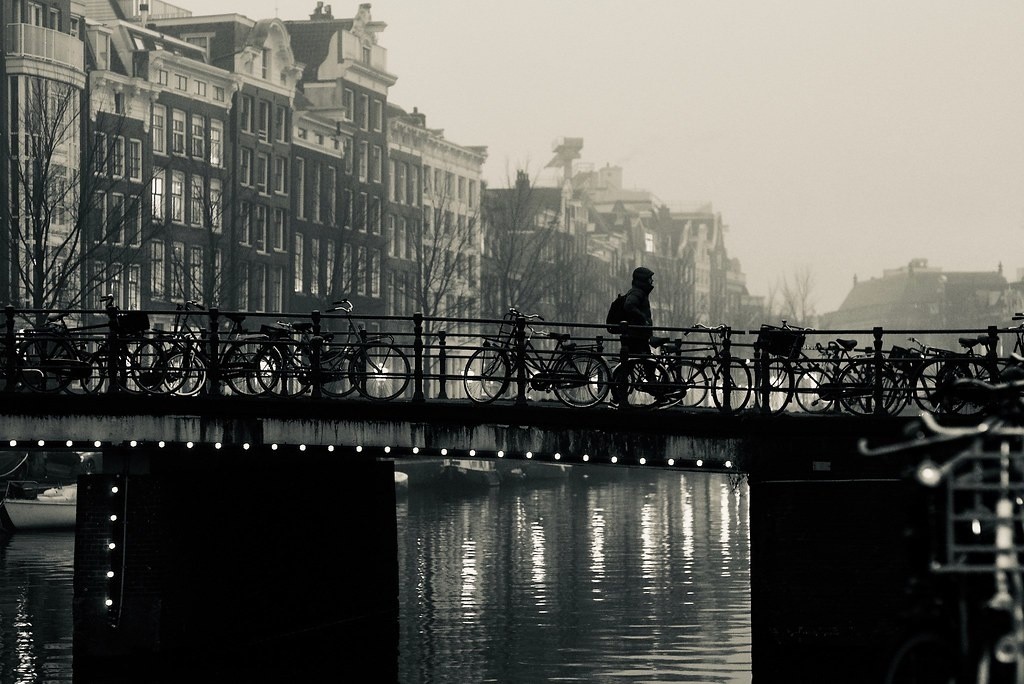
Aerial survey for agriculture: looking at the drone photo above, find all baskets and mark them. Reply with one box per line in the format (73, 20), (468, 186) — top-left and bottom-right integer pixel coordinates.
(887, 345), (909, 370)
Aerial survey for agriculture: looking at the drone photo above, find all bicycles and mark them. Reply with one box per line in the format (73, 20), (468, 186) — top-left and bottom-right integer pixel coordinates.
(463, 306), (752, 409)
(756, 311), (1024, 415)
(859, 377), (1024, 684)
(14, 293), (410, 400)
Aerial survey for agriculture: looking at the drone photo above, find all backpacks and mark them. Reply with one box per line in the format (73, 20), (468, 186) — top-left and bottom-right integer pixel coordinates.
(606, 290), (627, 335)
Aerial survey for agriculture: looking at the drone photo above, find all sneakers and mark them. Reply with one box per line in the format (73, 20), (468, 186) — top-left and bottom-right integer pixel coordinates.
(607, 398), (628, 411)
(657, 396), (681, 410)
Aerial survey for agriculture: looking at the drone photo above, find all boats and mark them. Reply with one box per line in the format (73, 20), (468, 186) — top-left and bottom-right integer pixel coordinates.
(1, 470), (78, 531)
(434, 463), (487, 502)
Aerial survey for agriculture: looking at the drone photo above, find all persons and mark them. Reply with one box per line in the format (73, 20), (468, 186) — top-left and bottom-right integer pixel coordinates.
(606, 267), (682, 410)
